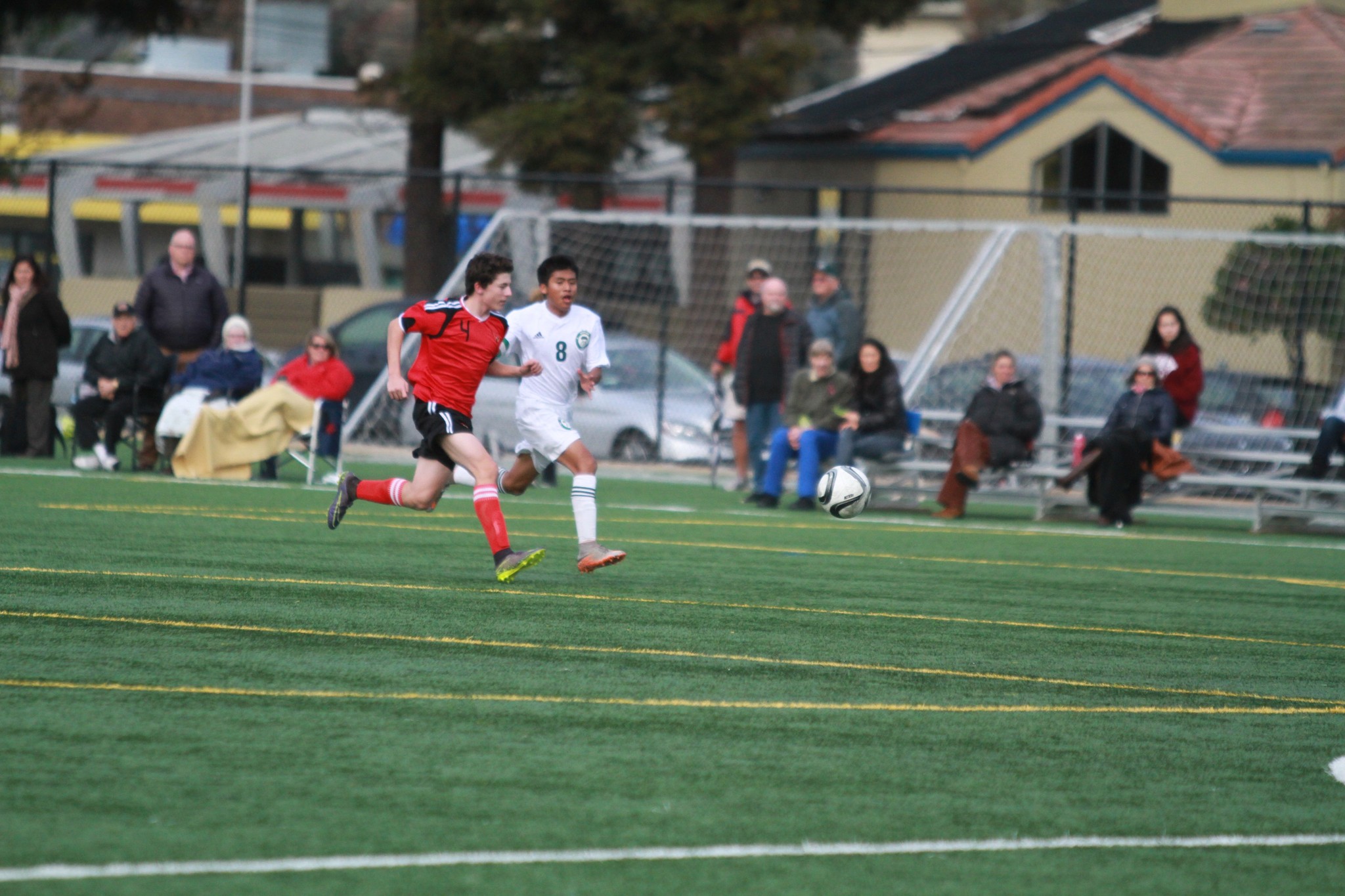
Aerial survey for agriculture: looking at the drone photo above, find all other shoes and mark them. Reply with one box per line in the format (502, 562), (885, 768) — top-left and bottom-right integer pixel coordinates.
(74, 455), (99, 471)
(954, 469), (978, 489)
(936, 508), (962, 519)
(755, 494), (775, 507)
(102, 458), (119, 470)
(1055, 476), (1072, 492)
(791, 498), (813, 510)
(1294, 464), (1327, 482)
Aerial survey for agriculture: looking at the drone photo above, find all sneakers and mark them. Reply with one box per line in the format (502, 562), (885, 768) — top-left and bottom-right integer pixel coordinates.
(327, 472), (356, 529)
(577, 545), (627, 573)
(496, 547), (545, 583)
(426, 489), (445, 513)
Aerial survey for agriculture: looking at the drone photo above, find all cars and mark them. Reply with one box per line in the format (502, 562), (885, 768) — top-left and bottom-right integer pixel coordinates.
(265, 295), (738, 465)
(0, 316), (116, 407)
(892, 351), (1344, 511)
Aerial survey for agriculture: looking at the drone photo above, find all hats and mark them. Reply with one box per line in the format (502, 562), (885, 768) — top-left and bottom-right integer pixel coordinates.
(816, 255), (844, 278)
(746, 259), (769, 278)
(113, 303), (134, 318)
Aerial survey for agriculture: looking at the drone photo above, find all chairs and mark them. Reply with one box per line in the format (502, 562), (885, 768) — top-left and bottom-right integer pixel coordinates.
(72, 380), (138, 469)
(286, 398), (344, 484)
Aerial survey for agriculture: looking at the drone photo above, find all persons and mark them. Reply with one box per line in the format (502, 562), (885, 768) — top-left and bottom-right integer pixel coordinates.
(329, 250), (546, 583)
(448, 254), (626, 574)
(1294, 392), (1345, 483)
(0, 227), (352, 483)
(712, 256), (1205, 530)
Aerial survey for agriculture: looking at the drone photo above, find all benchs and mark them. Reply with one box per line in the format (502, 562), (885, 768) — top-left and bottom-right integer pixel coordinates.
(856, 407), (1345, 531)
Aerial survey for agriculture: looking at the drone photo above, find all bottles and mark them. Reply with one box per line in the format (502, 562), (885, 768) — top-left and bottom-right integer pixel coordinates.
(1071, 432), (1086, 469)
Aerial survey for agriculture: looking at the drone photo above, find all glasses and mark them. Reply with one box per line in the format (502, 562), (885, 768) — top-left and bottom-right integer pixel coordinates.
(1135, 370), (1154, 376)
(312, 344), (332, 349)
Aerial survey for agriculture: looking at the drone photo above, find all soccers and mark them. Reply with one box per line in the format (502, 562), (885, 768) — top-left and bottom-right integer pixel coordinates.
(817, 465), (871, 519)
